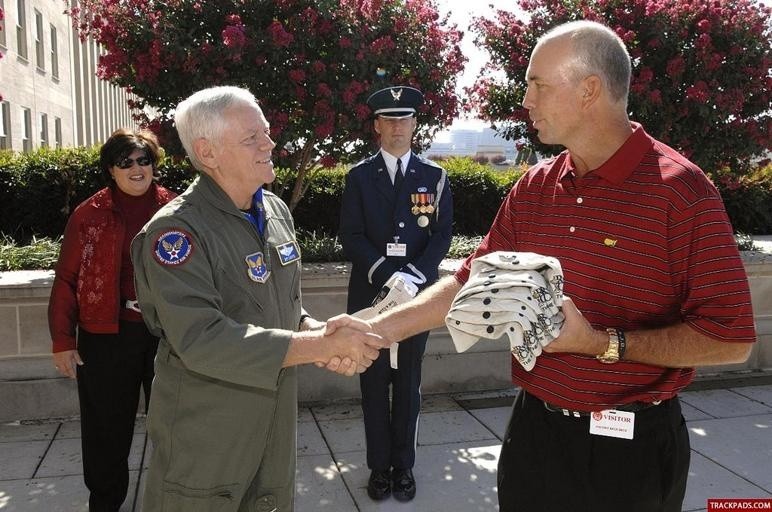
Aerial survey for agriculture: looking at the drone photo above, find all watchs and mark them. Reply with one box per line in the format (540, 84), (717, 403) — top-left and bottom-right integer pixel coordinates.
(597, 327), (618, 364)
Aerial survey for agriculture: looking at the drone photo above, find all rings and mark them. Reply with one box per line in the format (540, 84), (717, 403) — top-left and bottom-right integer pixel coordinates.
(55, 364), (58, 370)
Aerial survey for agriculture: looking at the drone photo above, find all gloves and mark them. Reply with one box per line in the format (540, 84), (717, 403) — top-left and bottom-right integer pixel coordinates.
(385, 271), (423, 297)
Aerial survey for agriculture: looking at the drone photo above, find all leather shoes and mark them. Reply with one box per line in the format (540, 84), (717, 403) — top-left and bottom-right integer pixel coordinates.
(369, 465), (391, 500)
(393, 464), (415, 500)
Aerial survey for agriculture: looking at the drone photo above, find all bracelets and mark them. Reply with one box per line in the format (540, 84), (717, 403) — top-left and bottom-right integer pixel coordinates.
(615, 327), (626, 362)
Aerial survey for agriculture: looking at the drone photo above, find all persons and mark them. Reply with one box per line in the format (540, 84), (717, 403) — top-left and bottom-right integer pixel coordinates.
(48, 128), (181, 511)
(131, 86), (393, 510)
(312, 21), (759, 511)
(343, 85), (454, 502)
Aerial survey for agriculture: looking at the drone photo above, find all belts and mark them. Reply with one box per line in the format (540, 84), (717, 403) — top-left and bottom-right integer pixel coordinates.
(541, 398), (662, 419)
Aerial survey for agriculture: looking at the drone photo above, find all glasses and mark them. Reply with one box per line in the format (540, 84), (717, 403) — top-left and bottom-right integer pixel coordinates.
(115, 157), (151, 169)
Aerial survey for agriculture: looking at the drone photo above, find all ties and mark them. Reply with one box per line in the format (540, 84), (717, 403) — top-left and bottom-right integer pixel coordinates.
(393, 158), (404, 192)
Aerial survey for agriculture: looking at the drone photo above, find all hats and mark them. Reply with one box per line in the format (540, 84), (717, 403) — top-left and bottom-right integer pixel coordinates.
(368, 86), (423, 120)
